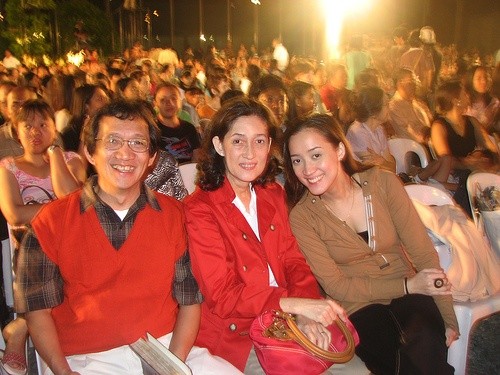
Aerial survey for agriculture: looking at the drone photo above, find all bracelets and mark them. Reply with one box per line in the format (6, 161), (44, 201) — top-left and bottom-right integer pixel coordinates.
(404, 277), (410, 295)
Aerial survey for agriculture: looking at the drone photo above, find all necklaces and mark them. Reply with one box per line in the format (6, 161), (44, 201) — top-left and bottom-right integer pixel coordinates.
(344, 180), (354, 223)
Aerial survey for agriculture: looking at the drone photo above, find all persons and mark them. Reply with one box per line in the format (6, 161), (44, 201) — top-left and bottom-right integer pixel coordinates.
(0, 21), (500, 375)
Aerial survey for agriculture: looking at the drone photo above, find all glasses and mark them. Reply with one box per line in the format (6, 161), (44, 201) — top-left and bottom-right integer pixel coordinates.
(95, 134), (152, 153)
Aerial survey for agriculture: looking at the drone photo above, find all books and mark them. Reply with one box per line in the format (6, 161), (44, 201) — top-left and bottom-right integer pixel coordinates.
(129, 331), (193, 375)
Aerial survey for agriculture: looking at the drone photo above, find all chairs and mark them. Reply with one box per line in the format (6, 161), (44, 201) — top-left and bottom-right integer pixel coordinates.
(388, 138), (500, 375)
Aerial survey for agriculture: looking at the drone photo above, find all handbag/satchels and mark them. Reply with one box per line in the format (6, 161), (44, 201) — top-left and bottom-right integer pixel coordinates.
(11, 185), (55, 270)
(249, 298), (360, 375)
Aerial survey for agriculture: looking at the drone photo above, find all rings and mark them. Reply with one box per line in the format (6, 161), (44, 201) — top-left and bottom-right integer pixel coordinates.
(320, 331), (326, 335)
(434, 278), (444, 288)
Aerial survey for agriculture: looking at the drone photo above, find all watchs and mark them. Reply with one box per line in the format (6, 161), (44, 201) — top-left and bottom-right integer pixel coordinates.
(46, 144), (62, 154)
